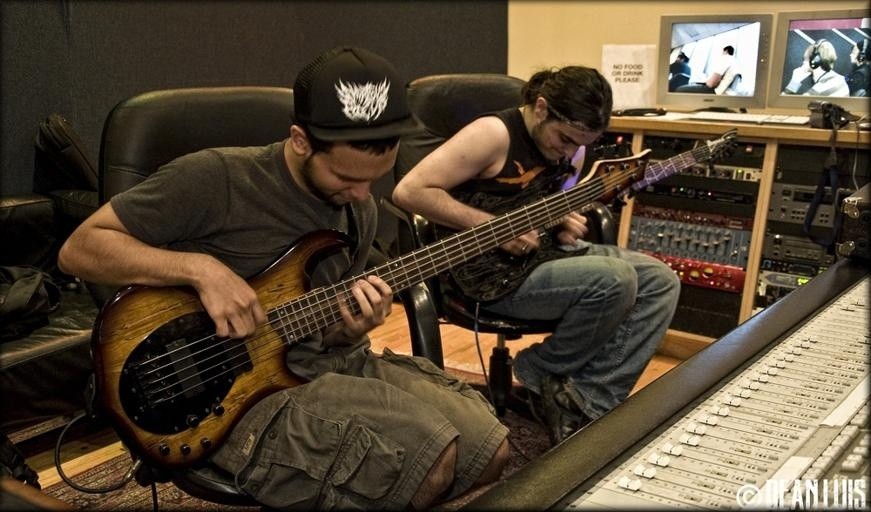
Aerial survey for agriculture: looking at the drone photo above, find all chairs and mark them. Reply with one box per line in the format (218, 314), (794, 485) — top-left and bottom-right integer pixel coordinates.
(90, 71), (563, 509)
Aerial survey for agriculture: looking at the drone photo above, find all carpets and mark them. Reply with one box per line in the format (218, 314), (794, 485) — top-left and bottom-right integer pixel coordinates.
(36, 343), (572, 508)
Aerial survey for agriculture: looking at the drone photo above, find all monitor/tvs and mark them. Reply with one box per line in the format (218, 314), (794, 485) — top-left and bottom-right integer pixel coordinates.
(656, 14), (773, 109)
(767, 9), (871, 112)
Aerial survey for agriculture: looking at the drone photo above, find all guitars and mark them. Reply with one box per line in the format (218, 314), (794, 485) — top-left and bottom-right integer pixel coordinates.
(91, 149), (652, 483)
(416, 128), (739, 304)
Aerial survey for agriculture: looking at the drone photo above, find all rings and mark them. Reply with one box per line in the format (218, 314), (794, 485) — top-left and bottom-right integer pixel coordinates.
(521, 242), (528, 251)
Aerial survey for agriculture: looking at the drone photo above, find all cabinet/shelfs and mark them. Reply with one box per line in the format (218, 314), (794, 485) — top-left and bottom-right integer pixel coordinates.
(598, 113), (871, 356)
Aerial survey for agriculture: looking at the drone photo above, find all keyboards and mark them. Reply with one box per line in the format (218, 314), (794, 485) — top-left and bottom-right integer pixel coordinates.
(689, 111), (810, 126)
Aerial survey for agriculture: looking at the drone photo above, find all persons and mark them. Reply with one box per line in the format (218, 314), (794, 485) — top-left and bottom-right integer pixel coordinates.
(57, 42), (511, 512)
(668, 46), (735, 94)
(391, 66), (680, 445)
(779, 38), (871, 97)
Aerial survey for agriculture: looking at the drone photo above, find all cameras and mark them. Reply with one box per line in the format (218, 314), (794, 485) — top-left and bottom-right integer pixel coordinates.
(807, 99), (842, 129)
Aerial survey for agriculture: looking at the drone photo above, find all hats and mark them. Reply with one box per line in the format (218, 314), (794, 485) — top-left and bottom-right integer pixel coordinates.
(294, 45), (421, 141)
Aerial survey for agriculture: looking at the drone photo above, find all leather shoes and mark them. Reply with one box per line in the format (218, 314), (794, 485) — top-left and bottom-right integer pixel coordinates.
(513, 362), (584, 446)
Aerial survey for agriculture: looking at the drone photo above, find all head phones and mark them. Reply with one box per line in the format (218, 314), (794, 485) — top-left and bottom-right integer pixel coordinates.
(858, 38), (869, 62)
(810, 38), (826, 69)
